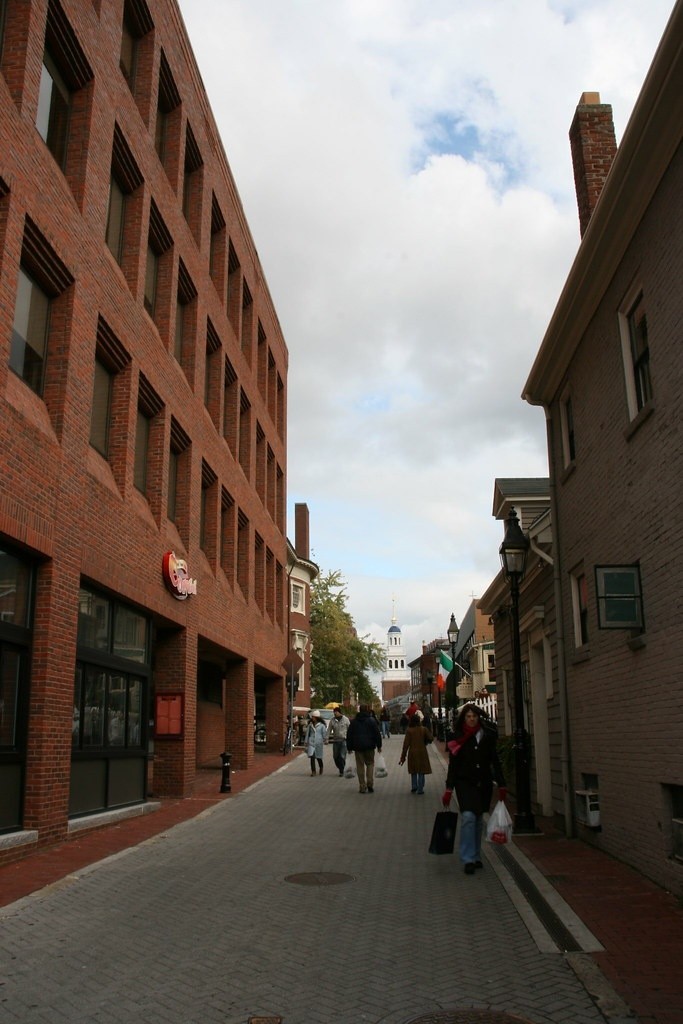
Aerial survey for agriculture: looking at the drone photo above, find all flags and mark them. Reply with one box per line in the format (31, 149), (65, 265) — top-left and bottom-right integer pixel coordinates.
(436, 650), (453, 692)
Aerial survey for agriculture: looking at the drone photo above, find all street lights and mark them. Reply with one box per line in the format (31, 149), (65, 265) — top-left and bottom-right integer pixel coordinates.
(498, 503), (543, 834)
(447, 613), (460, 730)
(433, 643), (446, 742)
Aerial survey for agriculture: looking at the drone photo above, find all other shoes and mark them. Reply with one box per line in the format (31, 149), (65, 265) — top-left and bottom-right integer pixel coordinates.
(411, 789), (417, 792)
(418, 791), (424, 794)
(368, 788), (374, 792)
(311, 770), (316, 776)
(464, 864), (476, 875)
(320, 767), (323, 774)
(476, 860), (483, 868)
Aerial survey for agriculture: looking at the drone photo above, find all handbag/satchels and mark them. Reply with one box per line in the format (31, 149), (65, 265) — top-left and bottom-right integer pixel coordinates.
(486, 798), (513, 843)
(344, 752), (356, 779)
(428, 805), (459, 855)
(374, 751), (388, 778)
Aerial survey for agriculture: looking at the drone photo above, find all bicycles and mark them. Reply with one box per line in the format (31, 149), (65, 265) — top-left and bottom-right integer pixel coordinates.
(284, 725), (299, 756)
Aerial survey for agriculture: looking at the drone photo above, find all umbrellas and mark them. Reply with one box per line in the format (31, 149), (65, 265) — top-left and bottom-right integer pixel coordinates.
(324, 702), (341, 709)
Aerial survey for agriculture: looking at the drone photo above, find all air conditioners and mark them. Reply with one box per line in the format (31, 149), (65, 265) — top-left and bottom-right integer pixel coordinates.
(575, 791), (600, 829)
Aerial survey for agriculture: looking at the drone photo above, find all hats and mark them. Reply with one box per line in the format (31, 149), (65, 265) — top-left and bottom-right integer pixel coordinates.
(312, 710), (320, 716)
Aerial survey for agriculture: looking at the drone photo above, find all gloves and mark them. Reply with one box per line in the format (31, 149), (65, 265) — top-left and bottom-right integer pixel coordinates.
(499, 788), (506, 801)
(443, 791), (452, 806)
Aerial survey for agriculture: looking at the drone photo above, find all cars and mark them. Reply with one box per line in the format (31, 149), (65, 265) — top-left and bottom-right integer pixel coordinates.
(307, 709), (336, 742)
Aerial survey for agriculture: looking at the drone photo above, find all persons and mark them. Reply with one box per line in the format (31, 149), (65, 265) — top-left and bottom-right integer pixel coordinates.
(304, 696), (433, 777)
(398, 715), (433, 796)
(346, 704), (382, 793)
(70, 701), (138, 746)
(442, 703), (508, 874)
